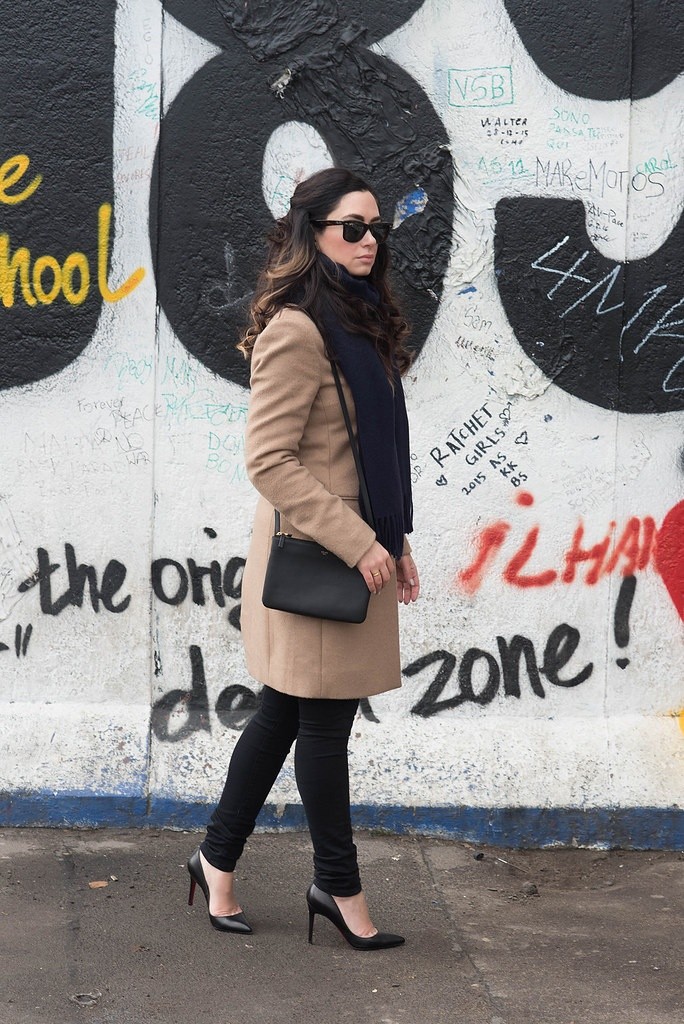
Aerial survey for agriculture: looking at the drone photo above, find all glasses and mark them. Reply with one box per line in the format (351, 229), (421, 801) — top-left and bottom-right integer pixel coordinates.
(311, 220), (393, 244)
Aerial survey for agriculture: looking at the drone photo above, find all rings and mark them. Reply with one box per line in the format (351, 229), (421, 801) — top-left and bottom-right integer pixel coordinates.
(372, 572), (381, 577)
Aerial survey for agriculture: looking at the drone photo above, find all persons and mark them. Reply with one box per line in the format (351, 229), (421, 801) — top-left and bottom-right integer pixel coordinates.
(188, 167), (420, 950)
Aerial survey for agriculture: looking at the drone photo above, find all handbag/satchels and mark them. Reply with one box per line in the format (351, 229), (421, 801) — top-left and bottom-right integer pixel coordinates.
(261, 531), (373, 623)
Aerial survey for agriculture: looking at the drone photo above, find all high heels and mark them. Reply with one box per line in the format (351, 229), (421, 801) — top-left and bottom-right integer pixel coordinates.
(306, 883), (405, 950)
(187, 847), (253, 934)
